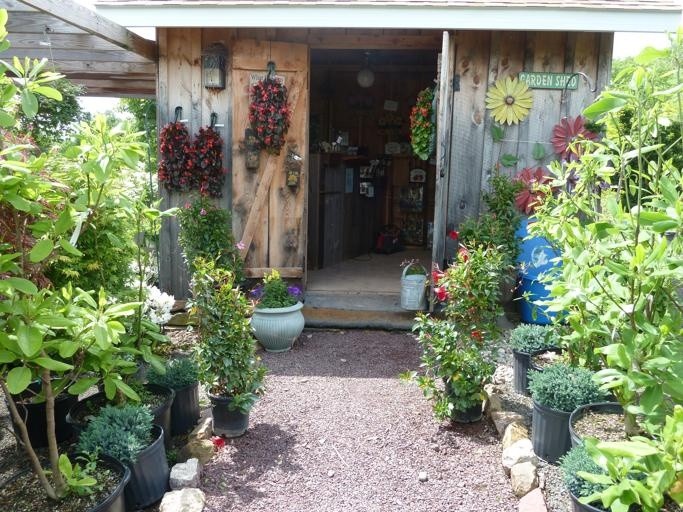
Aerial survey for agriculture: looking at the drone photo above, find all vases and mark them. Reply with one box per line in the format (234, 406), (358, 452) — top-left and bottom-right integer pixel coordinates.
(250, 300), (306, 353)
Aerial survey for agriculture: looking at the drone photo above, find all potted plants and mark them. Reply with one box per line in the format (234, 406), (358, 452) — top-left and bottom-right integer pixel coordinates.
(0, 10), (260, 512)
(409, 171), (682, 512)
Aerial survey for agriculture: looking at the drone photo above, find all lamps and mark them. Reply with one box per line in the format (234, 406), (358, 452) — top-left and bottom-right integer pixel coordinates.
(201, 42), (229, 89)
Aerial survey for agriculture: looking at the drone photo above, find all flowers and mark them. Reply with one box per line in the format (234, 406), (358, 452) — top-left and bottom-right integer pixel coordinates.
(249, 74), (290, 156)
(250, 270), (303, 308)
(410, 86), (434, 162)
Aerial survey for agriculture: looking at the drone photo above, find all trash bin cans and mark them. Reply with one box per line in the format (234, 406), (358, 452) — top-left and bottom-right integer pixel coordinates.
(514, 218), (570, 326)
